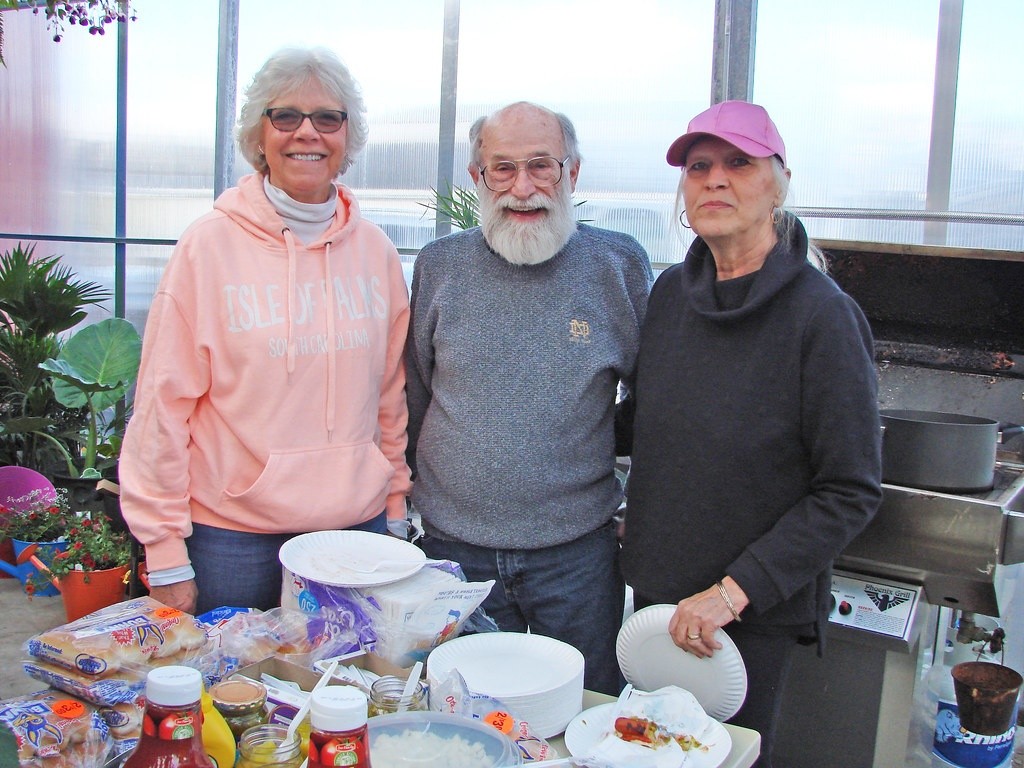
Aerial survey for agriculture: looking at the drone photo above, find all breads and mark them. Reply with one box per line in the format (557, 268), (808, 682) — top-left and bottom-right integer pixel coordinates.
(17, 603), (309, 768)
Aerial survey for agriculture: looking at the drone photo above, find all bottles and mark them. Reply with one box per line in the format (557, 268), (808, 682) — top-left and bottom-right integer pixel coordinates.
(208, 679), (270, 747)
(122, 665), (218, 768)
(233, 723), (305, 768)
(202, 682), (236, 768)
(368, 675), (426, 717)
(305, 685), (372, 768)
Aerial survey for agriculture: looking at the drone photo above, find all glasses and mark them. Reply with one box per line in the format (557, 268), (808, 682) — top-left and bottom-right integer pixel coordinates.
(479, 156), (572, 191)
(262, 107), (347, 133)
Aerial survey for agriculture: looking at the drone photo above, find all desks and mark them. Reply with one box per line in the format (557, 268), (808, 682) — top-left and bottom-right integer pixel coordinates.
(0, 603), (760, 768)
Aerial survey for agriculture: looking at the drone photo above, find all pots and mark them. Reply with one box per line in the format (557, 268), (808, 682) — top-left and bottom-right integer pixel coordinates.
(879, 409), (1024, 492)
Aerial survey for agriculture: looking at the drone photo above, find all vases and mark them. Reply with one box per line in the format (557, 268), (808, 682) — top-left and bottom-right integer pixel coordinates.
(57, 562), (131, 624)
(11, 538), (69, 597)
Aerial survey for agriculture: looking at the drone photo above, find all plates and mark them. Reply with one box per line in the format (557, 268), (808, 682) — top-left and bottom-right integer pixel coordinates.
(615, 604), (747, 724)
(424, 631), (584, 740)
(564, 700), (732, 768)
(278, 529), (427, 588)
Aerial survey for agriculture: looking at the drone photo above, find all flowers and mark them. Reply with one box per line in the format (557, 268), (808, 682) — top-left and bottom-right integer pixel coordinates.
(0, 489), (91, 543)
(24, 514), (141, 597)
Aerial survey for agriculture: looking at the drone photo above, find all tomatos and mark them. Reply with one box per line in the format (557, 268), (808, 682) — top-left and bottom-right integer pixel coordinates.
(159, 711), (199, 740)
(144, 713), (155, 736)
(308, 731), (366, 766)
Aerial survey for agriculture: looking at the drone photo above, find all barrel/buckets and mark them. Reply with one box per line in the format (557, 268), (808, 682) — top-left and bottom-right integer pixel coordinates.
(951, 631), (1024, 736)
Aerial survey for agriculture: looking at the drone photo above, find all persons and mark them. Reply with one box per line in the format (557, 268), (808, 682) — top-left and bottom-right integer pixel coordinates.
(613, 102), (883, 767)
(118, 47), (414, 613)
(404, 100), (653, 697)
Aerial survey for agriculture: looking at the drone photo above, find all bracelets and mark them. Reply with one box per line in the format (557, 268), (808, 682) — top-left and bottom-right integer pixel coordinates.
(717, 580), (742, 621)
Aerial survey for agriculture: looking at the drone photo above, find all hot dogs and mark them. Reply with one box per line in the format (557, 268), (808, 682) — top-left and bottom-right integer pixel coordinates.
(614, 716), (656, 743)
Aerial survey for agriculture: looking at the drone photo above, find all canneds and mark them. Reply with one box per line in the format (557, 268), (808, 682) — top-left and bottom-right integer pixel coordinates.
(207, 680), (307, 768)
(366, 676), (429, 718)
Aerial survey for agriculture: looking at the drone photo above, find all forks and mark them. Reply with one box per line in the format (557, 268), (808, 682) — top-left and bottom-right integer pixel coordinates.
(271, 661), (338, 762)
(341, 558), (447, 574)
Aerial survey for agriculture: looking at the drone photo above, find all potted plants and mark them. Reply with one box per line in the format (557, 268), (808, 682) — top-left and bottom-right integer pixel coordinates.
(0, 242), (142, 534)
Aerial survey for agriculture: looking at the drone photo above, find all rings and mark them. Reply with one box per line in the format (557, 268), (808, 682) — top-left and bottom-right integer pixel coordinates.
(687, 634), (699, 639)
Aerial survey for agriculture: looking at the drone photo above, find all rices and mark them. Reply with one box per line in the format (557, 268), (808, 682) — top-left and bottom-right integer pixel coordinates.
(369, 730), (497, 768)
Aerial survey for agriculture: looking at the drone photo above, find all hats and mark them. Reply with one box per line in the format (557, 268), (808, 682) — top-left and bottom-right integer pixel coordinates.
(667, 101), (787, 169)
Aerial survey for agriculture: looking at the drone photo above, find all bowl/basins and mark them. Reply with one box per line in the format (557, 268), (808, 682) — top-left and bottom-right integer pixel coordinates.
(299, 710), (522, 768)
